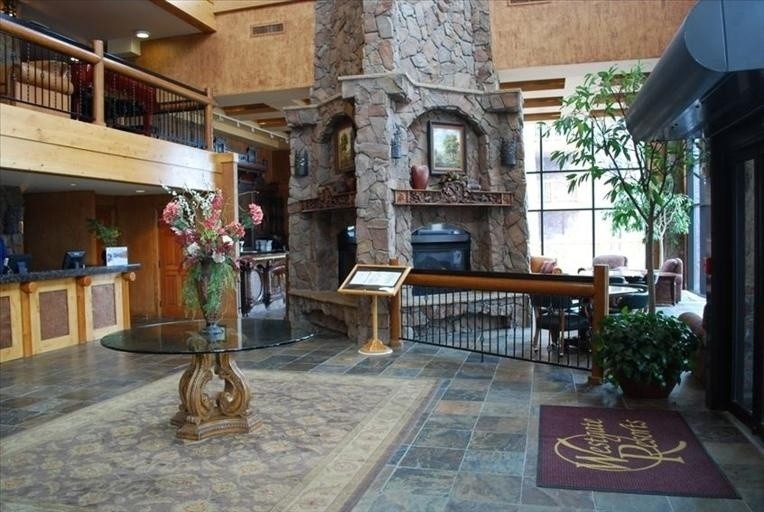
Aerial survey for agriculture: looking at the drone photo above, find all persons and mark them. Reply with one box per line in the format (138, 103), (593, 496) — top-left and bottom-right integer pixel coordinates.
(552, 268), (562, 274)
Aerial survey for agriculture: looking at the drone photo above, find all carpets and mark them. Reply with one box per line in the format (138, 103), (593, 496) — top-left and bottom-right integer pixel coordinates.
(537, 405), (743, 499)
(0, 367), (440, 511)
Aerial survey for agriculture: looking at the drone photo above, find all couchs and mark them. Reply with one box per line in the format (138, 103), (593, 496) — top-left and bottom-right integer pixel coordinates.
(0, 60), (75, 118)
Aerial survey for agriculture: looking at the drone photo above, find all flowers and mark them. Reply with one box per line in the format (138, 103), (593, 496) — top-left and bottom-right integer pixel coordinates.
(158, 173), (264, 313)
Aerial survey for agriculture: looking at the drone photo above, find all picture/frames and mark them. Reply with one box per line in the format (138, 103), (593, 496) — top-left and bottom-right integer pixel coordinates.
(427, 121), (468, 176)
(333, 122), (357, 175)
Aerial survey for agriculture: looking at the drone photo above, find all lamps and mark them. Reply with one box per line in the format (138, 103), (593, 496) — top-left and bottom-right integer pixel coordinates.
(0, 0), (18, 18)
(135, 30), (150, 39)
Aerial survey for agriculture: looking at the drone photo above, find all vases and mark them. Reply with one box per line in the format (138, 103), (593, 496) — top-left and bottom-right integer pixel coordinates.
(411, 166), (430, 190)
(196, 261), (225, 342)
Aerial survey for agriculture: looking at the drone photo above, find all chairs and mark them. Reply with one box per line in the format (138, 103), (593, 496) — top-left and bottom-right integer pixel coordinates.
(677, 312), (711, 388)
(521, 254), (683, 357)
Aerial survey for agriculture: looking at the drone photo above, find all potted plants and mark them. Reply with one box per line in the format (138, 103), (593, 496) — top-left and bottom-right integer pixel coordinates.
(585, 307), (701, 401)
(84, 218), (123, 266)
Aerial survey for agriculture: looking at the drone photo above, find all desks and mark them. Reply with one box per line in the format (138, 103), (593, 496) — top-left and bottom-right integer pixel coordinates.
(69, 63), (156, 136)
(101, 318), (318, 442)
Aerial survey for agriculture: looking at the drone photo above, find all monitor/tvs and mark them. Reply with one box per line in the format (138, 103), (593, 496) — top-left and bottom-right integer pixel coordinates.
(63, 250), (85, 268)
(8, 255), (34, 275)
